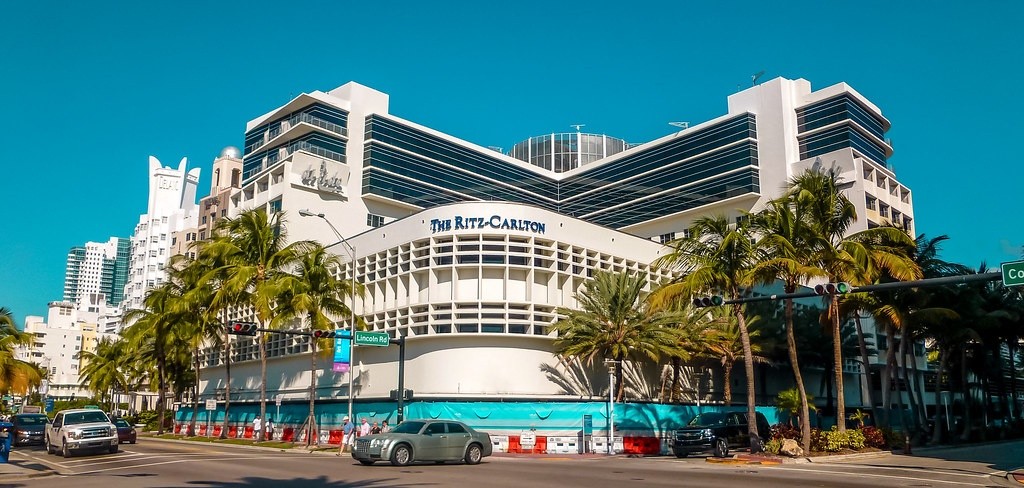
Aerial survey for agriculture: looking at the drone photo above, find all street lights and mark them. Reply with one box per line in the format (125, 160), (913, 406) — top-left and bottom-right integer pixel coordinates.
(602, 359), (622, 454)
(299, 208), (354, 451)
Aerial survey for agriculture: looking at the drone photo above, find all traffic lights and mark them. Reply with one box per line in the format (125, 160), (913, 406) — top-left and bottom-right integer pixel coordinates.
(227, 321), (258, 336)
(814, 283), (852, 295)
(691, 296), (726, 308)
(311, 329), (335, 338)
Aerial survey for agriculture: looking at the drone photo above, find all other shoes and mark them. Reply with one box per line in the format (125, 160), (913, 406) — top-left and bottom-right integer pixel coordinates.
(336, 453), (342, 457)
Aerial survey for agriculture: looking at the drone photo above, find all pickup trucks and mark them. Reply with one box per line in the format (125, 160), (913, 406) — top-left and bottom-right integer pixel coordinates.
(41, 408), (121, 460)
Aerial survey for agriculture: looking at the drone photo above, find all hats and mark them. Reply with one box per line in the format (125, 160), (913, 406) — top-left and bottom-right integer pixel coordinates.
(343, 416), (349, 420)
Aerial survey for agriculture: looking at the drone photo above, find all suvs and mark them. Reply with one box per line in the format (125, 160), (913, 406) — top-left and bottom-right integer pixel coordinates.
(671, 410), (771, 459)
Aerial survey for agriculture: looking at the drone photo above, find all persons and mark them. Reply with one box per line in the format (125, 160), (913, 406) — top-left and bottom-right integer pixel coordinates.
(336, 416), (389, 456)
(265, 418), (274, 440)
(252, 415), (261, 438)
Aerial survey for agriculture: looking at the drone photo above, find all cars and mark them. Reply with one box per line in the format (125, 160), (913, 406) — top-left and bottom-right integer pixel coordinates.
(351, 419), (493, 466)
(111, 418), (137, 443)
(9, 413), (53, 447)
